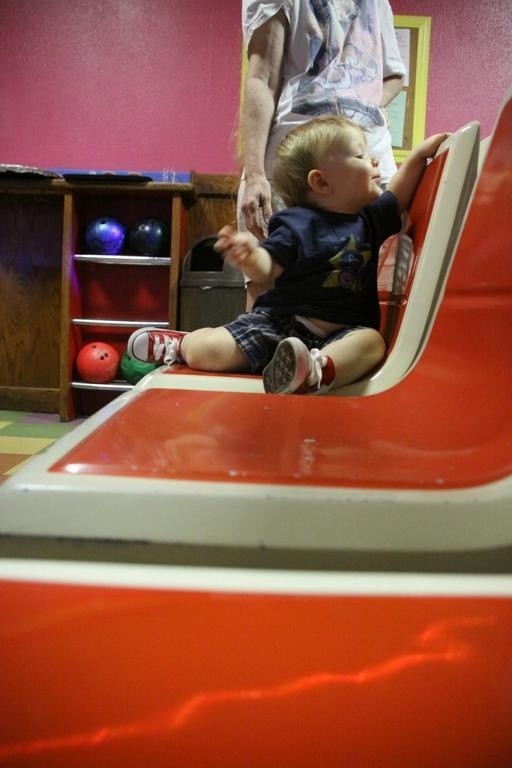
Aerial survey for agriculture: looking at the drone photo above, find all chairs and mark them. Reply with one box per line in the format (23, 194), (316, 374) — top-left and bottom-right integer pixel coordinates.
(0, 87), (512, 768)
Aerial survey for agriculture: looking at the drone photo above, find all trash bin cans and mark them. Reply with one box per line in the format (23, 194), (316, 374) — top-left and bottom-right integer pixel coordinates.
(179, 233), (247, 333)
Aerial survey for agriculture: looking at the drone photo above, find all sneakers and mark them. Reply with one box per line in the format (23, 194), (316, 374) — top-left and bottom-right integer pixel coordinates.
(127, 327), (189, 366)
(263, 336), (328, 394)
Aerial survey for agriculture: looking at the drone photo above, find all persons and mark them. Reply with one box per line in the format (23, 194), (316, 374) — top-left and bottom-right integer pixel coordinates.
(123, 112), (459, 396)
(234, 1), (408, 319)
